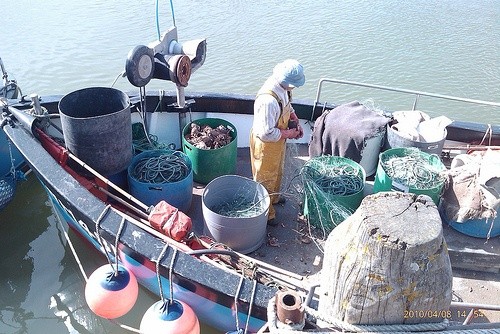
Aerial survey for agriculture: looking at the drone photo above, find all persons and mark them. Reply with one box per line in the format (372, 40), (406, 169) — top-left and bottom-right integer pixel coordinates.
(249, 60), (305, 225)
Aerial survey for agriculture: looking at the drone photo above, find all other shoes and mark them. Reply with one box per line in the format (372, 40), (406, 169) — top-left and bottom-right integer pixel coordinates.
(278, 195), (285, 203)
(267, 218), (279, 226)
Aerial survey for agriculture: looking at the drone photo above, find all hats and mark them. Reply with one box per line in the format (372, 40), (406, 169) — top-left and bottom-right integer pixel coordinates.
(273, 59), (305, 87)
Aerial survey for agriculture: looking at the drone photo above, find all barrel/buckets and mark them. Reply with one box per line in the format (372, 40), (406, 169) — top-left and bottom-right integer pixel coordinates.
(58, 87), (135, 177)
(181, 119), (238, 185)
(374, 147), (448, 207)
(201, 175), (272, 254)
(440, 176), (500, 238)
(386, 113), (447, 159)
(129, 147), (195, 212)
(302, 154), (365, 232)
(357, 133), (385, 178)
